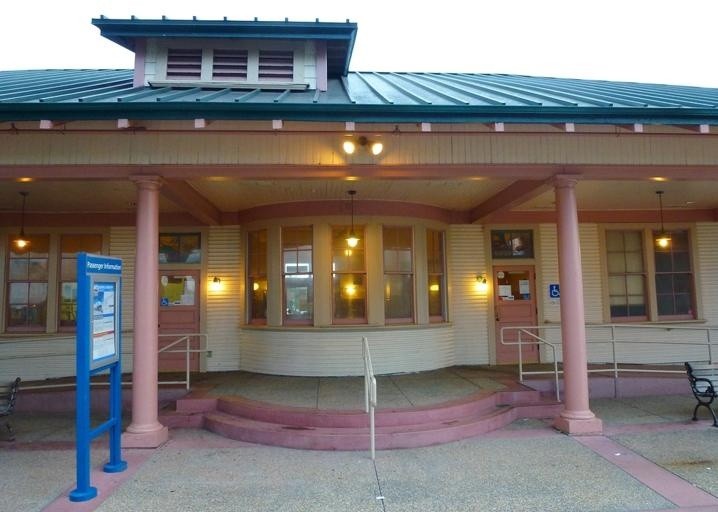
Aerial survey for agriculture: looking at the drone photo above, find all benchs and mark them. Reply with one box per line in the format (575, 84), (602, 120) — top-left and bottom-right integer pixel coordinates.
(684, 360), (718, 427)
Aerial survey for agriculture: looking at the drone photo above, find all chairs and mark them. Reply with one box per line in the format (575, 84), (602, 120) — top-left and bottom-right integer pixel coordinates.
(0, 376), (21, 442)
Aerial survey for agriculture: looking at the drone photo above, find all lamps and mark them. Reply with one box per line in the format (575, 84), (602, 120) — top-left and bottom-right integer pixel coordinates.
(12, 192), (30, 249)
(342, 137), (384, 155)
(345, 190), (360, 247)
(655, 191), (671, 248)
